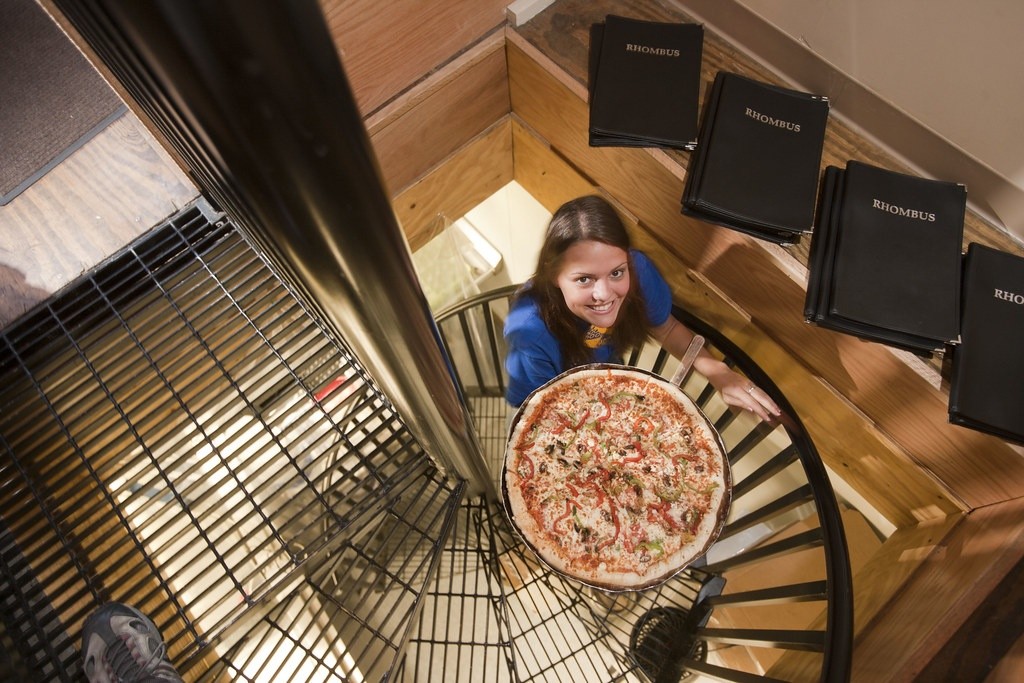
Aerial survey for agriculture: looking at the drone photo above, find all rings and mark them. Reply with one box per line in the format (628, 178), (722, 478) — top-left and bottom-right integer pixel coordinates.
(748, 387), (755, 393)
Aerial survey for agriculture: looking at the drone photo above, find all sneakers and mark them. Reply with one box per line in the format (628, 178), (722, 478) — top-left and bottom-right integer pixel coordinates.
(77, 602), (186, 683)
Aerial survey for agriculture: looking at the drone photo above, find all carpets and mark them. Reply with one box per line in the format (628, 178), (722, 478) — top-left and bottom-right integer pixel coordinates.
(0, 0), (120, 207)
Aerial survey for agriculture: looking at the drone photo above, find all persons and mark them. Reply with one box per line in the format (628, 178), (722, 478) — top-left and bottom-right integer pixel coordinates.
(80, 602), (179, 683)
(503, 195), (780, 424)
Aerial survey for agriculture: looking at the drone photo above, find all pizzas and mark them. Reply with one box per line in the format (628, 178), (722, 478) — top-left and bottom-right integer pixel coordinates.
(506, 369), (724, 586)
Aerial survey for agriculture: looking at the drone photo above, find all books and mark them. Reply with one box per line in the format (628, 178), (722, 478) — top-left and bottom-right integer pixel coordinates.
(587, 13), (1023, 444)
